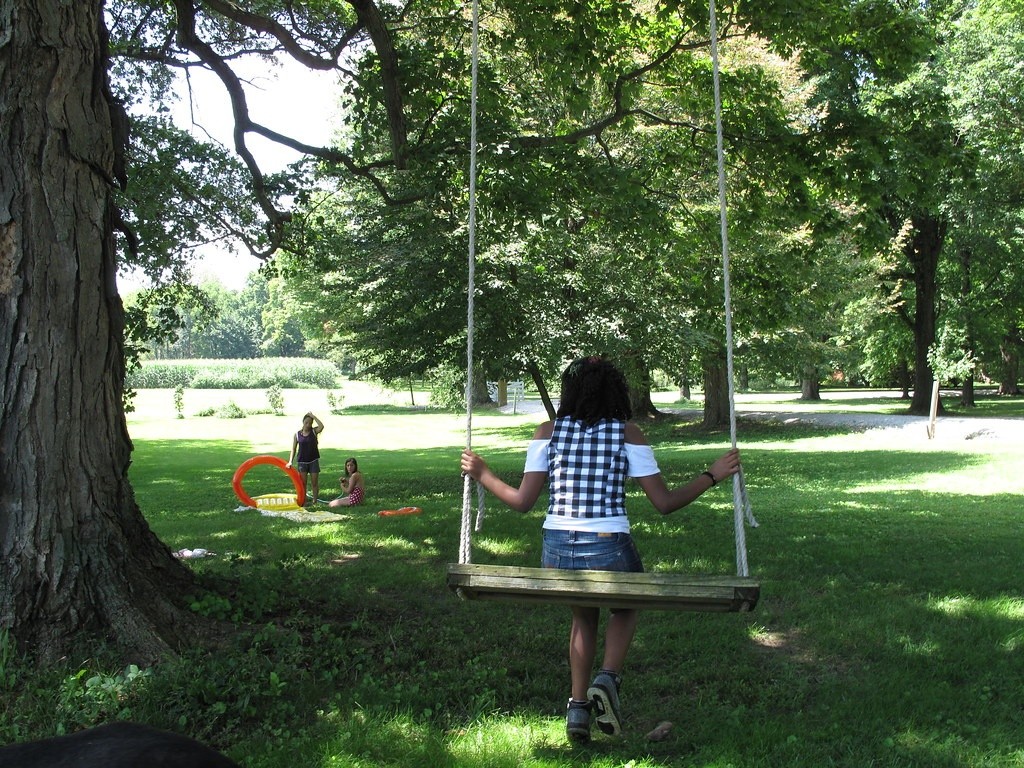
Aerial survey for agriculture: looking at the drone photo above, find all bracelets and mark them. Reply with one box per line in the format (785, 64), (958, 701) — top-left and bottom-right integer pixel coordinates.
(702, 471), (717, 486)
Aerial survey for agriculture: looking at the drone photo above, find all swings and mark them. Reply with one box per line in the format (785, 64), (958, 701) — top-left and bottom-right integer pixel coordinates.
(446, 0), (762, 615)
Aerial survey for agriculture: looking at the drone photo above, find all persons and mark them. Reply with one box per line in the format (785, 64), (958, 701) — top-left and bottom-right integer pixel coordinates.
(460, 356), (741, 739)
(287, 411), (324, 507)
(328, 458), (364, 509)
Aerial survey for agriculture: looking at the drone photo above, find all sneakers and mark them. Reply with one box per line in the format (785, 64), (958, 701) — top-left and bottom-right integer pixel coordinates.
(587, 670), (621, 737)
(566, 700), (592, 746)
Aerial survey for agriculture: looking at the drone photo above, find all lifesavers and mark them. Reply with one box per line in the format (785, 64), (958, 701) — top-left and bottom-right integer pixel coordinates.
(378, 506), (421, 516)
